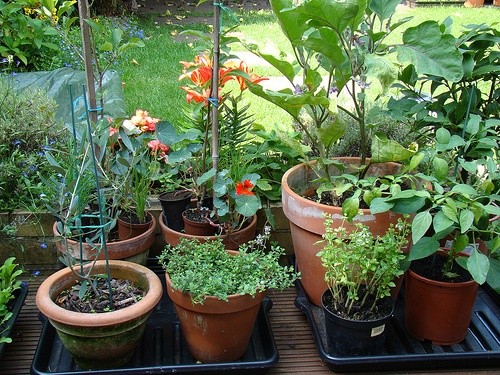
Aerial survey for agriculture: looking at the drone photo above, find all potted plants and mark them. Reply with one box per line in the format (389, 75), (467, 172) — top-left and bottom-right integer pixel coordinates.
(0, 0), (500, 375)
